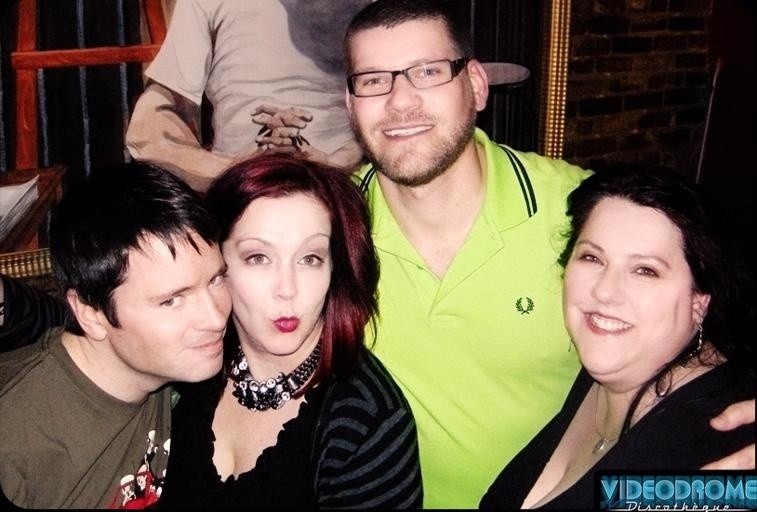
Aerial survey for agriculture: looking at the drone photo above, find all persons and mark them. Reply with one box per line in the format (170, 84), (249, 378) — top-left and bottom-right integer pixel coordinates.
(341, 1), (755, 511)
(0, 158), (232, 510)
(151, 148), (424, 511)
(473, 151), (756, 510)
(127, 0), (363, 195)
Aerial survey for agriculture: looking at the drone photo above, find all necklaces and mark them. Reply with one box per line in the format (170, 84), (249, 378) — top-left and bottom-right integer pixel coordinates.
(592, 345), (716, 456)
(227, 340), (329, 412)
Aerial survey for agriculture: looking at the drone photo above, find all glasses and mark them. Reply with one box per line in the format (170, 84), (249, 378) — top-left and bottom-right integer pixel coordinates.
(347, 57), (469, 98)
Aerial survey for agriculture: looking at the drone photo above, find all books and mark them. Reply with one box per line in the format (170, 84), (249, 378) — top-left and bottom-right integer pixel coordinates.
(0, 173), (42, 243)
